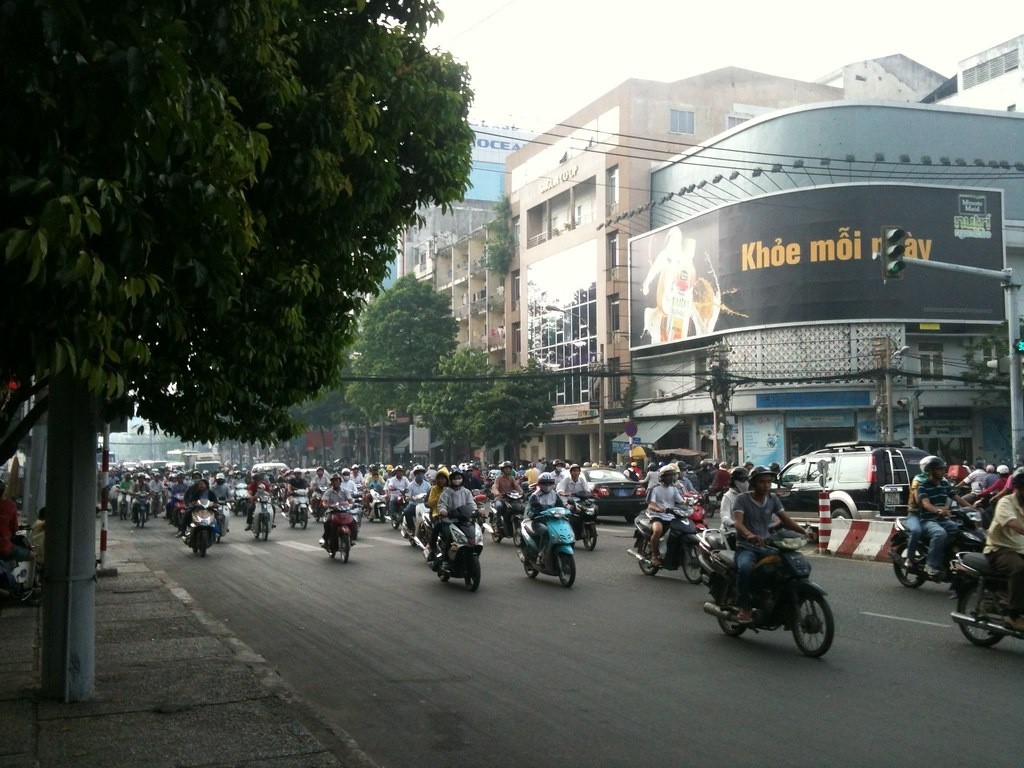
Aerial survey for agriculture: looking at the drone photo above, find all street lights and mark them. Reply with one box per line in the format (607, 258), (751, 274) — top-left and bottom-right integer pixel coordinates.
(545, 305), (590, 376)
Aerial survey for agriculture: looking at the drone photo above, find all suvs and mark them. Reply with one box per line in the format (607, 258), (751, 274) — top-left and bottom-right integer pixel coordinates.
(769, 438), (937, 520)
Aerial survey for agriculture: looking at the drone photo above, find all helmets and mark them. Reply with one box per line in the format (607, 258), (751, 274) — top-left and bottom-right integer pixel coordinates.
(106, 463), (477, 479)
(1013, 466), (1024, 487)
(552, 458), (781, 482)
(986, 465), (994, 473)
(500, 461), (512, 470)
(538, 472), (556, 484)
(919, 455), (947, 474)
(997, 465), (1009, 474)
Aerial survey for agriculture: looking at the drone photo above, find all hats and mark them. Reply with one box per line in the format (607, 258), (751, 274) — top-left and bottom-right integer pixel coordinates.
(259, 483), (266, 489)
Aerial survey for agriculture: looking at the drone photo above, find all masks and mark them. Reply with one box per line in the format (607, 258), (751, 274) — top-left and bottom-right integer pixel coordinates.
(451, 478), (463, 486)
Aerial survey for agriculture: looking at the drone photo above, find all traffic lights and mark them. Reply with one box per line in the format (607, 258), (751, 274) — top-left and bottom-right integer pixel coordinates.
(880, 225), (905, 282)
(1014, 339), (1024, 353)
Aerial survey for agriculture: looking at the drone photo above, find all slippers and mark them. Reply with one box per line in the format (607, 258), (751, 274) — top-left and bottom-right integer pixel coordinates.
(737, 613), (753, 623)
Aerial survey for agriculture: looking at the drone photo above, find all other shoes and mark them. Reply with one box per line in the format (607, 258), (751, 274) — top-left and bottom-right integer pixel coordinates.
(245, 525), (252, 531)
(427, 551), (436, 561)
(536, 554), (542, 564)
(924, 563), (939, 575)
(905, 559), (913, 568)
(175, 531), (184, 538)
(652, 558), (662, 567)
(1003, 615), (1024, 632)
(1000, 595), (1010, 606)
(495, 525), (500, 532)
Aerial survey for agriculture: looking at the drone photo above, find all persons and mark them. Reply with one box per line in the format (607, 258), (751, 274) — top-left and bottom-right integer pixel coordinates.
(245, 473), (276, 530)
(734, 465), (817, 623)
(554, 463), (592, 510)
(943, 455), (1024, 527)
(721, 469), (750, 541)
(0, 480), (46, 588)
(917, 458), (984, 577)
(646, 465), (687, 566)
(985, 465), (1024, 632)
(321, 473), (353, 546)
(287, 467), (310, 520)
(210, 473), (233, 502)
(490, 461), (524, 535)
(389, 464), (410, 521)
(904, 456), (937, 567)
(436, 468), (479, 554)
(97, 458), (781, 569)
(525, 472), (567, 565)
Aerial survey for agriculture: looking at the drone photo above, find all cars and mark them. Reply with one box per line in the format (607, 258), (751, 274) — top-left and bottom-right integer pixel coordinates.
(95, 449), (296, 481)
(579, 466), (648, 522)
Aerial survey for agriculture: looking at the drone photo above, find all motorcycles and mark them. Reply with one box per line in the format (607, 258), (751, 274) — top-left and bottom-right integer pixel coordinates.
(948, 550), (1024, 648)
(517, 506), (577, 589)
(885, 491), (996, 589)
(972, 482), (996, 530)
(562, 491), (600, 551)
(680, 491), (710, 533)
(627, 507), (705, 584)
(97, 475), (530, 592)
(695, 529), (836, 658)
